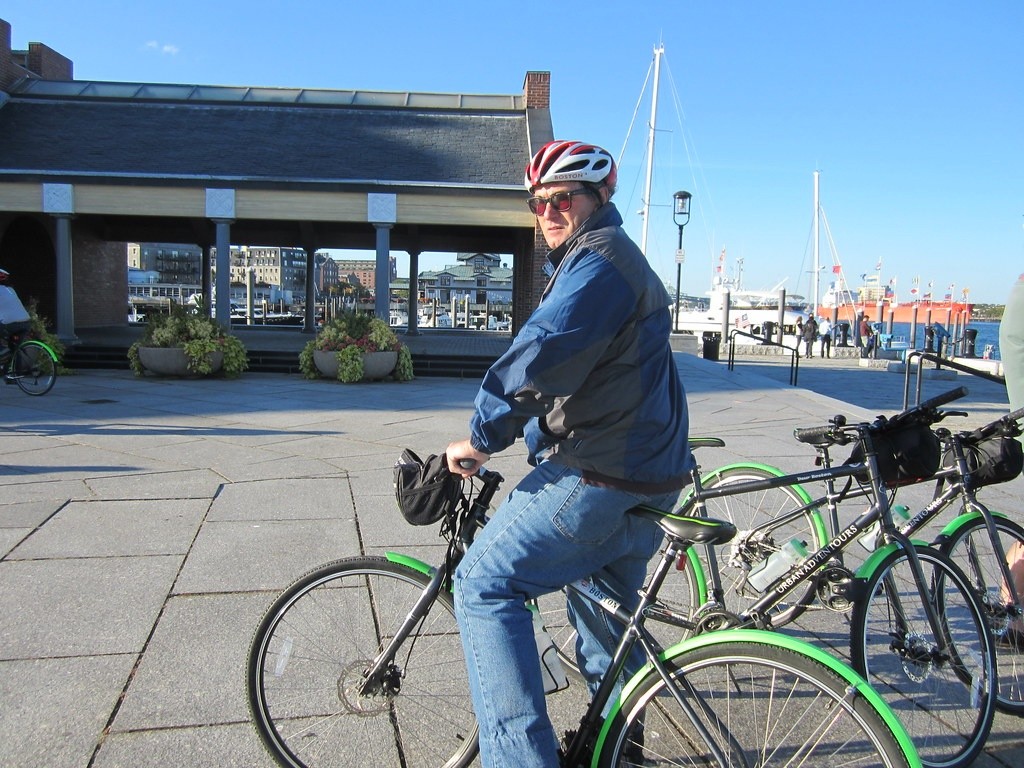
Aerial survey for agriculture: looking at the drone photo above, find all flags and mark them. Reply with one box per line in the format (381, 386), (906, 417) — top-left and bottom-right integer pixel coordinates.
(860, 274), (866, 281)
(875, 262), (882, 270)
(947, 284), (954, 290)
(833, 265), (842, 275)
(923, 291), (931, 298)
(909, 288), (919, 294)
(927, 281), (933, 287)
(944, 293), (953, 300)
(717, 266), (722, 272)
(911, 276), (919, 285)
(888, 278), (896, 286)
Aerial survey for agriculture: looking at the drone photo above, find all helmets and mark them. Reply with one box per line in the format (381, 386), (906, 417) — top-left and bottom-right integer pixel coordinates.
(0, 269), (10, 280)
(522, 140), (617, 193)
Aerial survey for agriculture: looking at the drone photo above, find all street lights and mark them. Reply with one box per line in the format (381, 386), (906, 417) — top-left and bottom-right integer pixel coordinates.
(674, 190), (692, 330)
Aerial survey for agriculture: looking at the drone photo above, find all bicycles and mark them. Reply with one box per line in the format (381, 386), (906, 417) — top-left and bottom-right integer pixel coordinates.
(681, 406), (1024, 715)
(0, 327), (58, 397)
(646, 384), (999, 768)
(247, 448), (1024, 768)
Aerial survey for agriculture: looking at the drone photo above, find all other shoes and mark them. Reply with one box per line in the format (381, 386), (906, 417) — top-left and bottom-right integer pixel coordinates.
(806, 355), (808, 358)
(810, 355), (812, 358)
(623, 733), (644, 768)
(796, 355), (802, 358)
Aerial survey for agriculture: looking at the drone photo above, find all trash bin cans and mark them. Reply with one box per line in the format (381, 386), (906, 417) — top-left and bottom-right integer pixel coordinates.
(702, 331), (722, 361)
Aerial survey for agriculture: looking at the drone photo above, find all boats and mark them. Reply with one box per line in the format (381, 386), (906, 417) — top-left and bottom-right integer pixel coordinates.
(715, 245), (808, 311)
(389, 305), (509, 330)
(791, 169), (912, 351)
(816, 256), (976, 325)
(616, 27), (824, 335)
(209, 301), (322, 325)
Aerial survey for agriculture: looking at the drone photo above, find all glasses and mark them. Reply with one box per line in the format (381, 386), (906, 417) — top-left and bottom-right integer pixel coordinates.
(525, 187), (596, 216)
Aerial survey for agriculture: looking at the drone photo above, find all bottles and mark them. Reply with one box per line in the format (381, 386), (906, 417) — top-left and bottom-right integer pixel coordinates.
(746, 539), (808, 592)
(859, 505), (910, 552)
(525, 605), (566, 694)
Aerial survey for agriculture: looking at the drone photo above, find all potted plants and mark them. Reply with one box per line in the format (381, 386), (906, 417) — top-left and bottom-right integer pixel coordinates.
(127, 296), (248, 381)
(0, 305), (82, 376)
(298, 310), (415, 384)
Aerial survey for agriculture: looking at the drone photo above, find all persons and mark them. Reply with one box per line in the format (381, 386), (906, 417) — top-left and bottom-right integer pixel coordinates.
(795, 312), (832, 359)
(445, 140), (697, 768)
(0, 268), (32, 379)
(860, 315), (874, 358)
(990, 272), (1024, 649)
(735, 313), (750, 328)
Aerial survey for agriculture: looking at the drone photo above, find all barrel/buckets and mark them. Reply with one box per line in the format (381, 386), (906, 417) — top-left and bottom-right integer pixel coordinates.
(703, 338), (718, 360)
